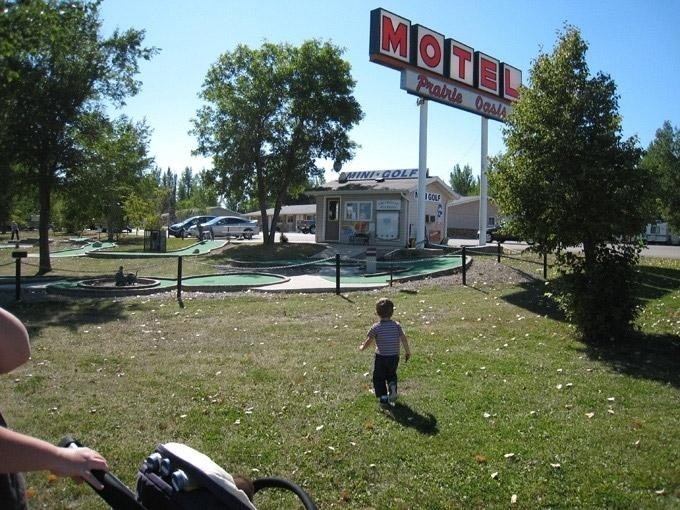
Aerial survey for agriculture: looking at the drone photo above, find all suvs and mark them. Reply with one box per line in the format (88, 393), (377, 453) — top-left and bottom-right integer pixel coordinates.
(475, 222), (526, 244)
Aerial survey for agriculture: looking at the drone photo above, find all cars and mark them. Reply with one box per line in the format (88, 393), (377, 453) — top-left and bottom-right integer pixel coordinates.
(188, 215), (261, 241)
(168, 215), (217, 237)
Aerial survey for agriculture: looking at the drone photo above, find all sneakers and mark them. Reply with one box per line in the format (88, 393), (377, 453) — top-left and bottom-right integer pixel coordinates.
(379, 397), (389, 407)
(388, 381), (398, 401)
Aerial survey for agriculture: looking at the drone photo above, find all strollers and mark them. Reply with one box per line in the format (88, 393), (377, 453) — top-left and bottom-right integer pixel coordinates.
(60, 432), (319, 510)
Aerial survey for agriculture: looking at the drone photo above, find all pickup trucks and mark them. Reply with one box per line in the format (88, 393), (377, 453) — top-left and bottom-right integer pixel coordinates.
(298, 218), (315, 234)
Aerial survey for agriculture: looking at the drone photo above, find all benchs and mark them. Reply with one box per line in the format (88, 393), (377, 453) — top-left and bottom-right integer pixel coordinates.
(348, 233), (369, 246)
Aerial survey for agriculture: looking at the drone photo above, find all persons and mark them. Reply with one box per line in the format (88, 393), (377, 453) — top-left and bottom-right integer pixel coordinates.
(358, 298), (410, 403)
(10, 220), (20, 240)
(0, 307), (109, 510)
(115, 266), (127, 286)
(125, 272), (137, 285)
